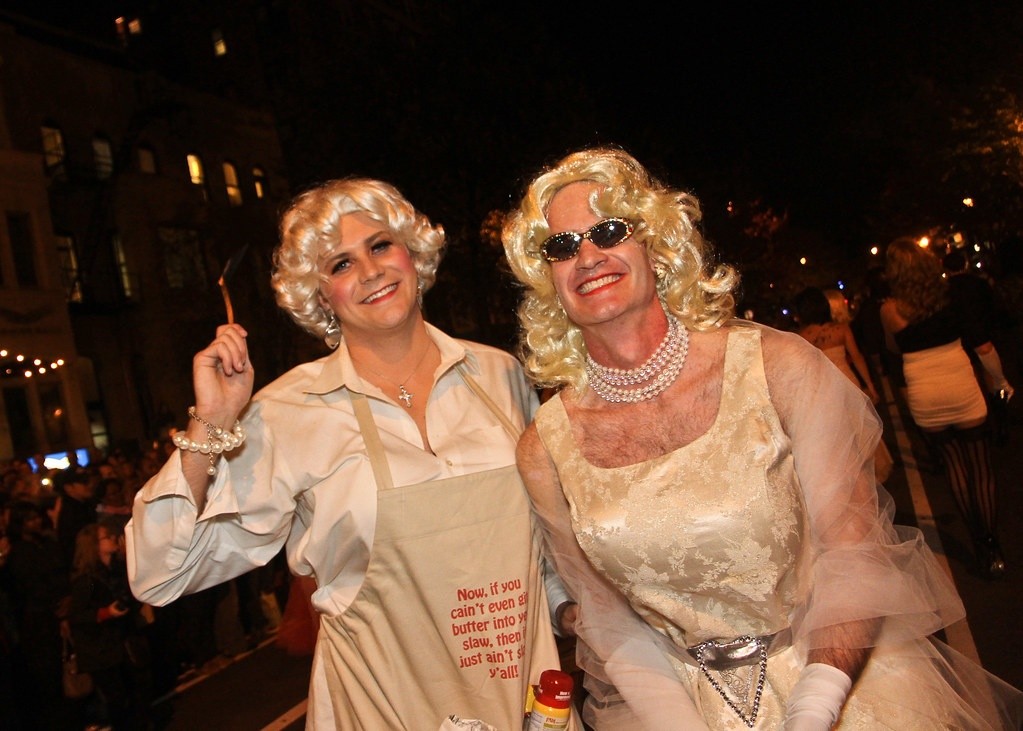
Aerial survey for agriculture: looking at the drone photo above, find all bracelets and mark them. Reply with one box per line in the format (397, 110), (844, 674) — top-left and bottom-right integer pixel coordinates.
(171, 406), (246, 476)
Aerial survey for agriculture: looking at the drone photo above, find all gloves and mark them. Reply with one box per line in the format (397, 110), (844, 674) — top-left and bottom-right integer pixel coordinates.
(603, 635), (708, 731)
(780, 662), (852, 731)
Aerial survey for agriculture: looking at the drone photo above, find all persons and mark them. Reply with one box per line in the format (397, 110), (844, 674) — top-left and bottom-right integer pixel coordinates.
(0, 438), (320, 731)
(790, 236), (1015, 575)
(500, 148), (1023, 730)
(121, 177), (584, 731)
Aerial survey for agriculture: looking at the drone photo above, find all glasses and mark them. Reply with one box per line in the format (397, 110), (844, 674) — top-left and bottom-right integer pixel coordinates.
(540, 216), (645, 263)
(100, 533), (110, 541)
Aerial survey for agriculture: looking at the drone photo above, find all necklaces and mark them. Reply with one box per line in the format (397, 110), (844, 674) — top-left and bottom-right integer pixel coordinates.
(586, 313), (689, 403)
(350, 340), (432, 408)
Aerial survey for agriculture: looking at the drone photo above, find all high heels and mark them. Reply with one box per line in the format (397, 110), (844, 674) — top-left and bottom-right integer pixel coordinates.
(973, 535), (1007, 580)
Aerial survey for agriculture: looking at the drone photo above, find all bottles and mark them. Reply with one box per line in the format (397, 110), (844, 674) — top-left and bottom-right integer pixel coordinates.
(528, 669), (574, 731)
